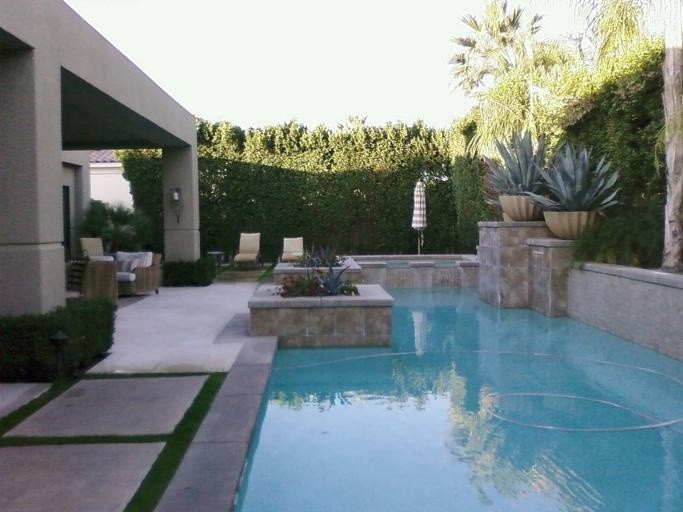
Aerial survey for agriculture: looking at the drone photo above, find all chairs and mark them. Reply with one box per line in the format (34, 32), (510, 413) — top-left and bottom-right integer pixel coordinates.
(79, 237), (114, 262)
(234, 233), (263, 270)
(282, 236), (304, 264)
(113, 251), (161, 296)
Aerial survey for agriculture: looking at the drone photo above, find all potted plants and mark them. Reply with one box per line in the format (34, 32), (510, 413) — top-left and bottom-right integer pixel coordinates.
(519, 139), (624, 240)
(481, 129), (557, 222)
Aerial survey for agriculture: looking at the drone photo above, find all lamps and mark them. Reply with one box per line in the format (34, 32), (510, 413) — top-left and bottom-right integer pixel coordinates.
(168, 187), (185, 222)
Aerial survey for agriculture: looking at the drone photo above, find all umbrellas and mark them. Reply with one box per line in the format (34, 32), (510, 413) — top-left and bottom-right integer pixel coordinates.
(412, 179), (427, 255)
